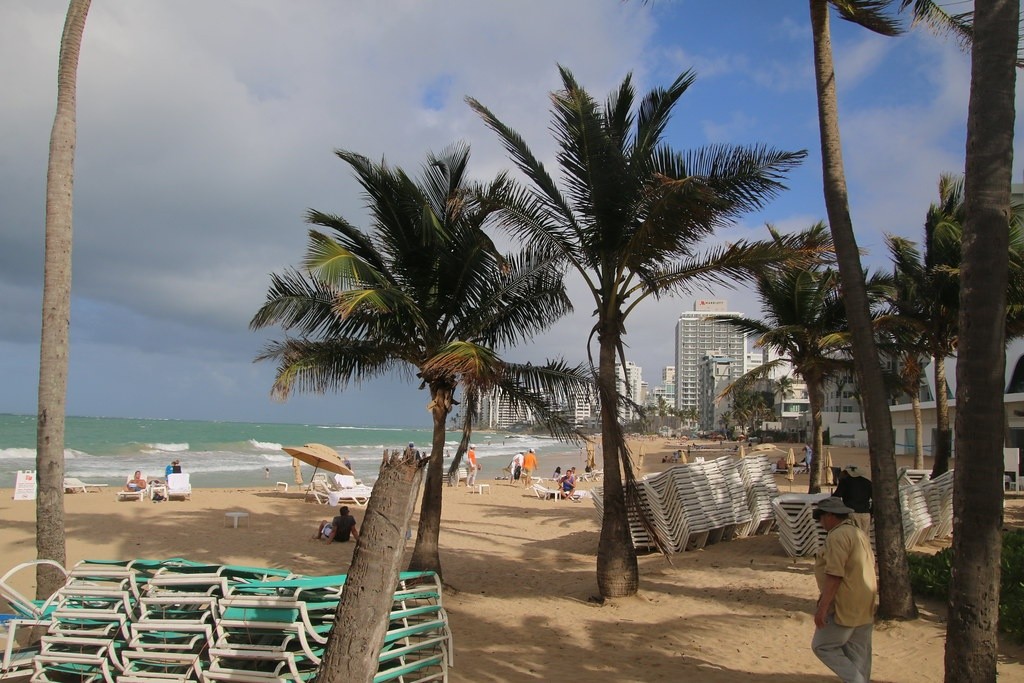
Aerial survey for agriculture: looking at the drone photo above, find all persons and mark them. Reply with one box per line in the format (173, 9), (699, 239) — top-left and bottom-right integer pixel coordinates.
(315, 507), (358, 544)
(553, 465), (577, 482)
(662, 444), (691, 463)
(558, 470), (577, 501)
(510, 448), (538, 487)
(265, 467), (271, 480)
(123, 471), (146, 492)
(810, 496), (879, 683)
(465, 444), (478, 486)
(165, 460), (181, 481)
(404, 442), (421, 460)
(488, 441), (491, 446)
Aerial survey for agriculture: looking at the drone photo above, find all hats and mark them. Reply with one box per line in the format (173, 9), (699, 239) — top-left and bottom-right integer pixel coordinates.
(812, 496), (854, 520)
(470, 444), (479, 449)
(529, 448), (535, 453)
(840, 466), (866, 478)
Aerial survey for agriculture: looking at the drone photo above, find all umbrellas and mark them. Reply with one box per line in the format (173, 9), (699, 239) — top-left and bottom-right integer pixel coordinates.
(739, 445), (745, 461)
(787, 448), (795, 493)
(281, 442), (356, 500)
(824, 449), (834, 492)
(637, 440), (646, 477)
(585, 436), (595, 482)
(681, 452), (687, 465)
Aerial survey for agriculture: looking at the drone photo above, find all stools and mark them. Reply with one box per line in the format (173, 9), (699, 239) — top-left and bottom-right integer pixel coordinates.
(224, 512), (249, 528)
(472, 484), (491, 495)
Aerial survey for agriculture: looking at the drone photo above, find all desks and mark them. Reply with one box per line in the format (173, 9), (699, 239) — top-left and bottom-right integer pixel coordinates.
(545, 491), (562, 501)
(150, 483), (166, 500)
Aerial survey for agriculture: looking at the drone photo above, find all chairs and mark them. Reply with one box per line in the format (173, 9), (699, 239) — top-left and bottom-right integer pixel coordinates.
(505, 445), (1024, 555)
(166, 473), (192, 501)
(117, 471), (150, 501)
(0, 558), (454, 683)
(64, 478), (108, 494)
(442, 468), (469, 487)
(307, 472), (373, 506)
